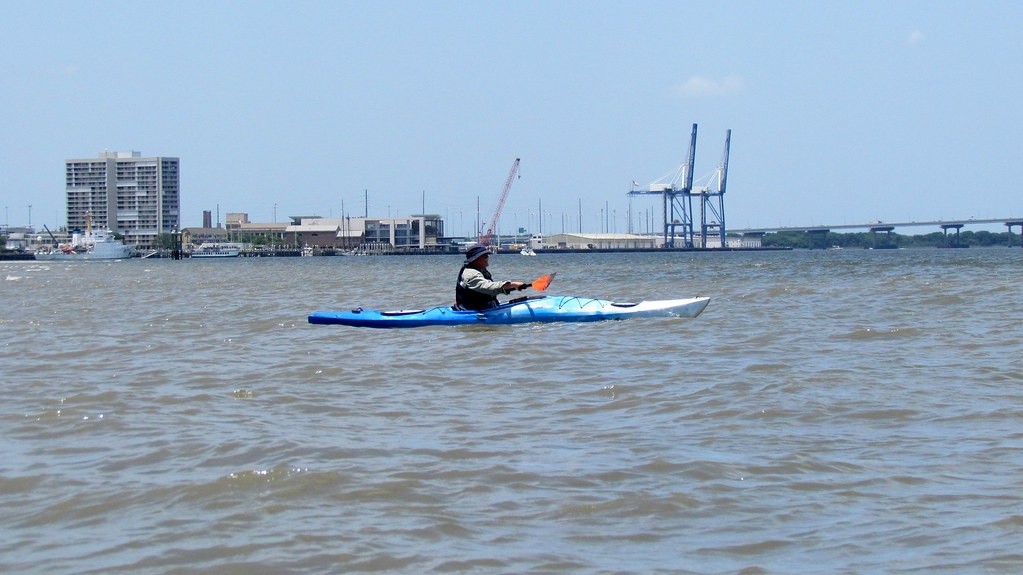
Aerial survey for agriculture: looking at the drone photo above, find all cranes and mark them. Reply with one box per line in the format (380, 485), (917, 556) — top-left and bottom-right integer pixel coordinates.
(626, 123), (731, 248)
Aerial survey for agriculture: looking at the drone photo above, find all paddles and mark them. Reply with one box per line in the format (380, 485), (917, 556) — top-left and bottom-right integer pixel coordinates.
(505, 272), (558, 292)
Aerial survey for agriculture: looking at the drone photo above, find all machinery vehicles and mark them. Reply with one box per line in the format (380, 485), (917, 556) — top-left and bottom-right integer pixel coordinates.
(480, 157), (521, 245)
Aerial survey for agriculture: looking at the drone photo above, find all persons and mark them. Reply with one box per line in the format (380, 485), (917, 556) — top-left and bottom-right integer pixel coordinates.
(456, 246), (524, 310)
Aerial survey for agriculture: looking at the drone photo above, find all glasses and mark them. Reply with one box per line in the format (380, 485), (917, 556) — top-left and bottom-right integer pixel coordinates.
(484, 255), (489, 259)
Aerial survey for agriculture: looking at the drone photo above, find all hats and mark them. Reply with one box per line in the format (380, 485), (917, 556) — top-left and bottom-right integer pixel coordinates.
(464, 245), (493, 263)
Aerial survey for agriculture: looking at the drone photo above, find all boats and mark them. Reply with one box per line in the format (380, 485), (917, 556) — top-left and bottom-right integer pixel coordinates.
(0, 224), (135, 261)
(307, 296), (711, 328)
(519, 249), (536, 257)
(191, 244), (241, 258)
